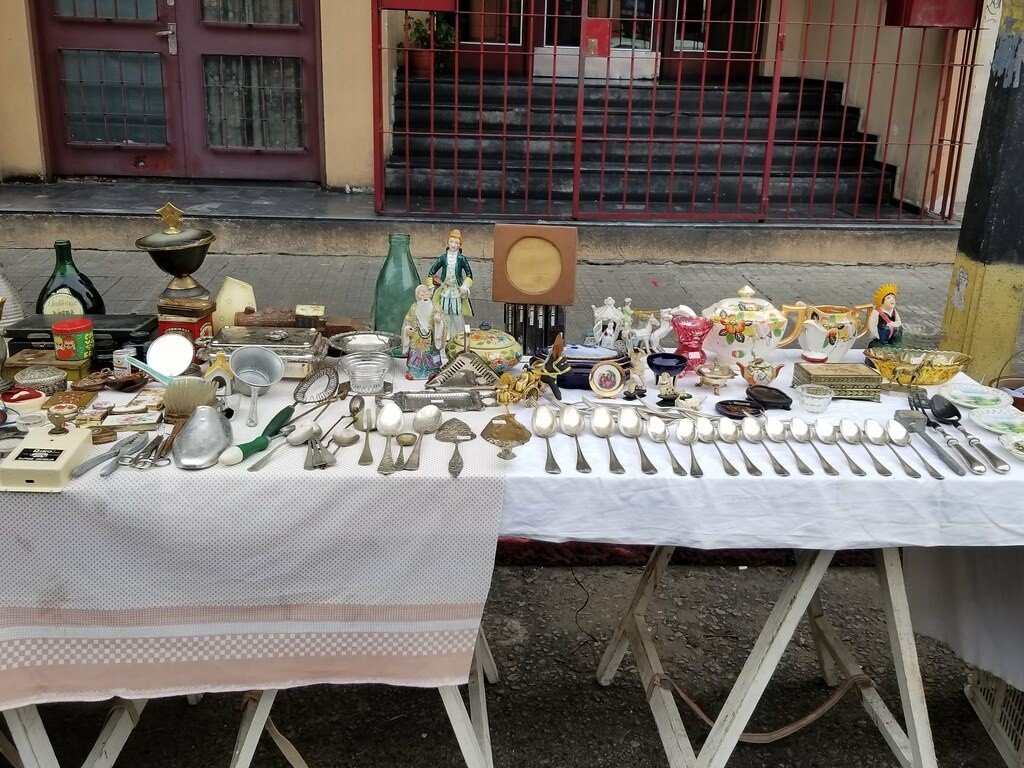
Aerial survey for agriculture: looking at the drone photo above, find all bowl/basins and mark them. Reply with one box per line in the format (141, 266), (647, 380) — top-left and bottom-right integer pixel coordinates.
(863, 347), (973, 386)
(329, 331), (403, 353)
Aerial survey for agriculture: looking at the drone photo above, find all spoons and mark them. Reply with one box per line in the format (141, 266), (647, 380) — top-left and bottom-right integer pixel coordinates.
(247, 394), (1012, 479)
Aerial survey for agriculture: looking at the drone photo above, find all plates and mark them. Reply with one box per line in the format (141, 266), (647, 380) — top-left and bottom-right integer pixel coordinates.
(968, 408), (1024, 435)
(938, 383), (1014, 408)
(998, 426), (1024, 461)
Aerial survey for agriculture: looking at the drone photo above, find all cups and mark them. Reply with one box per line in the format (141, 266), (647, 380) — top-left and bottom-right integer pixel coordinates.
(795, 301), (874, 361)
(341, 351), (391, 394)
(671, 315), (713, 369)
(795, 384), (835, 413)
(702, 284), (807, 365)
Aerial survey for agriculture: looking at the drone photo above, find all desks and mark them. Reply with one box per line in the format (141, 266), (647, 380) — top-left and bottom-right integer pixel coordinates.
(0, 344), (1024, 768)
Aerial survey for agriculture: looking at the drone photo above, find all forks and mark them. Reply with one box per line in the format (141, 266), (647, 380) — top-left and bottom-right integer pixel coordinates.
(907, 393), (986, 474)
(526, 393), (841, 432)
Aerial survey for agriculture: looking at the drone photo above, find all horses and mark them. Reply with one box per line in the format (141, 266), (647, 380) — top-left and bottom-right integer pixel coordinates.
(631, 308), (674, 353)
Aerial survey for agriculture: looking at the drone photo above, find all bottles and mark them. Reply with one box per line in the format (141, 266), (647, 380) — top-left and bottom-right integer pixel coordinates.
(36, 239), (105, 314)
(375, 232), (422, 358)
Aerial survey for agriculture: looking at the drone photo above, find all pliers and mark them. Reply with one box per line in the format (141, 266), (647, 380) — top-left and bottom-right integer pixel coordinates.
(71, 429), (150, 477)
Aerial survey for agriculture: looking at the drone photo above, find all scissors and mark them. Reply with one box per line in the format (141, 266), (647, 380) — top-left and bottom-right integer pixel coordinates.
(118, 434), (172, 470)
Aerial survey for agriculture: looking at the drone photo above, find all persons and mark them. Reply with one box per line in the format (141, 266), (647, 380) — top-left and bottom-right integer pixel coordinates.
(425, 229), (473, 340)
(401, 284), (447, 380)
(864, 283), (902, 369)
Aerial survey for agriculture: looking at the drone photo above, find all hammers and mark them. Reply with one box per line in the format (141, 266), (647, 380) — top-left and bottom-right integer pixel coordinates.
(893, 409), (967, 477)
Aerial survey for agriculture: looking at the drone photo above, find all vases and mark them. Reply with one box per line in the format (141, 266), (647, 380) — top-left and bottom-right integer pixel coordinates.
(671, 315), (714, 371)
